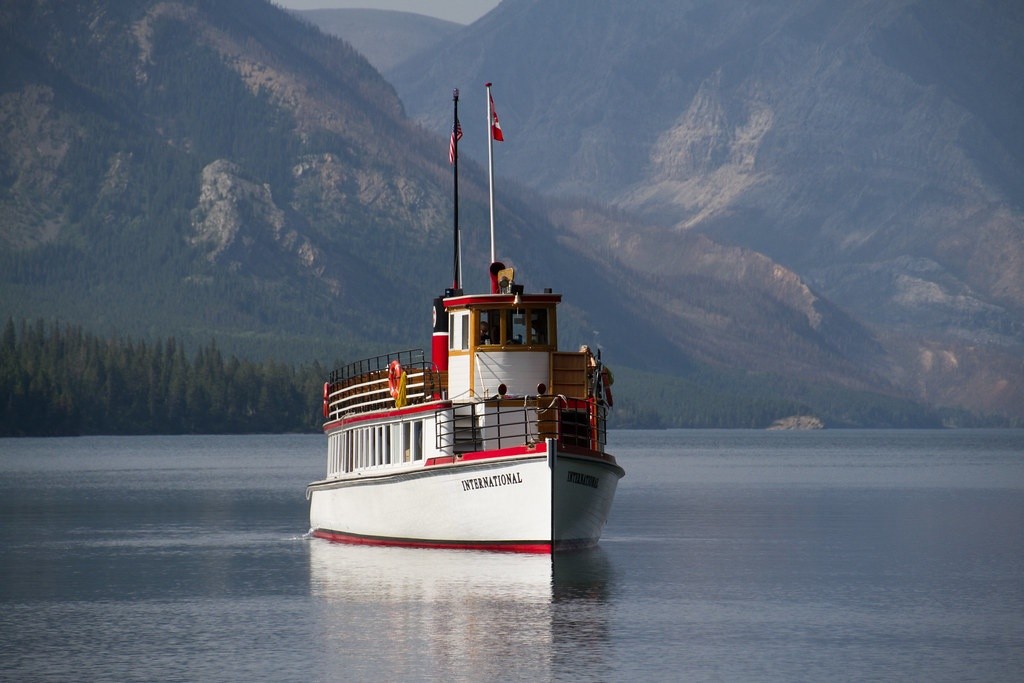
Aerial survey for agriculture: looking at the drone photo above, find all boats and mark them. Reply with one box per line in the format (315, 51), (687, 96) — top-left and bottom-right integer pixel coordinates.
(306, 83), (626, 554)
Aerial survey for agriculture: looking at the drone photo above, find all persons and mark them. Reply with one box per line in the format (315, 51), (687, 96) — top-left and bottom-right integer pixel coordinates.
(477, 319), (493, 344)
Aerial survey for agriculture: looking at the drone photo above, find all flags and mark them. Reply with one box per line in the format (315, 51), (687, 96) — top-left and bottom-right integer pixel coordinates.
(448, 112), (464, 163)
(485, 93), (504, 142)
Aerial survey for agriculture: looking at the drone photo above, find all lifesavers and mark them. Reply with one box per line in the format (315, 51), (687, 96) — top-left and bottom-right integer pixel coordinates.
(322, 381), (329, 418)
(388, 359), (403, 398)
